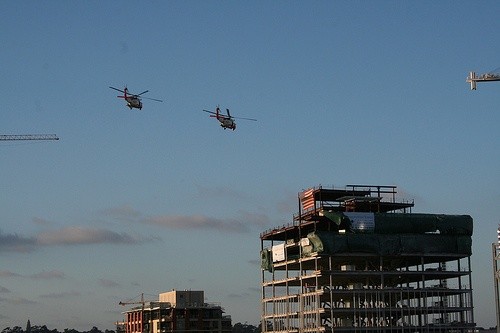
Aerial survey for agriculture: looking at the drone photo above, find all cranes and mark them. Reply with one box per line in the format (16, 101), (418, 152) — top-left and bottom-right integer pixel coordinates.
(118, 292), (159, 306)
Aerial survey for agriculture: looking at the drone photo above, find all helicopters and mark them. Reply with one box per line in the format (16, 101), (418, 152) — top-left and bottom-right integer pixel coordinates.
(202, 103), (258, 131)
(109, 83), (164, 111)
